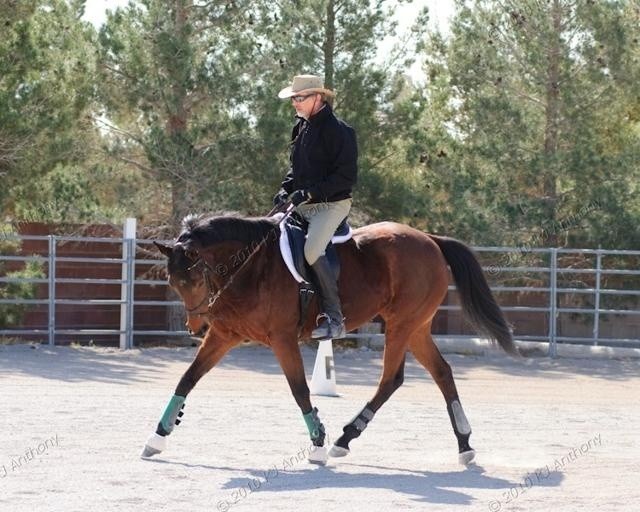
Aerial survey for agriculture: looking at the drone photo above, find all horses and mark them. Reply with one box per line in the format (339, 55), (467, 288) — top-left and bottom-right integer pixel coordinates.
(140, 209), (534, 465)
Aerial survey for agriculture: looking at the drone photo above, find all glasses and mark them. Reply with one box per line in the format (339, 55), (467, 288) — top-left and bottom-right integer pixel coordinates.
(291, 94), (314, 101)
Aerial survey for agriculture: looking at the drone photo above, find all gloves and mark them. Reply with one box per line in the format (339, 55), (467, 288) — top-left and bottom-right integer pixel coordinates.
(272, 189), (288, 203)
(289, 189), (311, 206)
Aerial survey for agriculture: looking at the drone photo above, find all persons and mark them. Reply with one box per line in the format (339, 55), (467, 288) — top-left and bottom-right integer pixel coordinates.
(272, 72), (361, 341)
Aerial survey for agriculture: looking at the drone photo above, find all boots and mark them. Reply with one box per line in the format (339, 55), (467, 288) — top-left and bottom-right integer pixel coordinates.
(310, 255), (346, 339)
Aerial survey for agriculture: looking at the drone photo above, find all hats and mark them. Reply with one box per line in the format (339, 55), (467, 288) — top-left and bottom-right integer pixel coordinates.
(277, 74), (336, 98)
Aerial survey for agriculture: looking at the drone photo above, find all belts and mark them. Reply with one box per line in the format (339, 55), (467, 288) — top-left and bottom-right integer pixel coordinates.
(308, 193), (352, 204)
